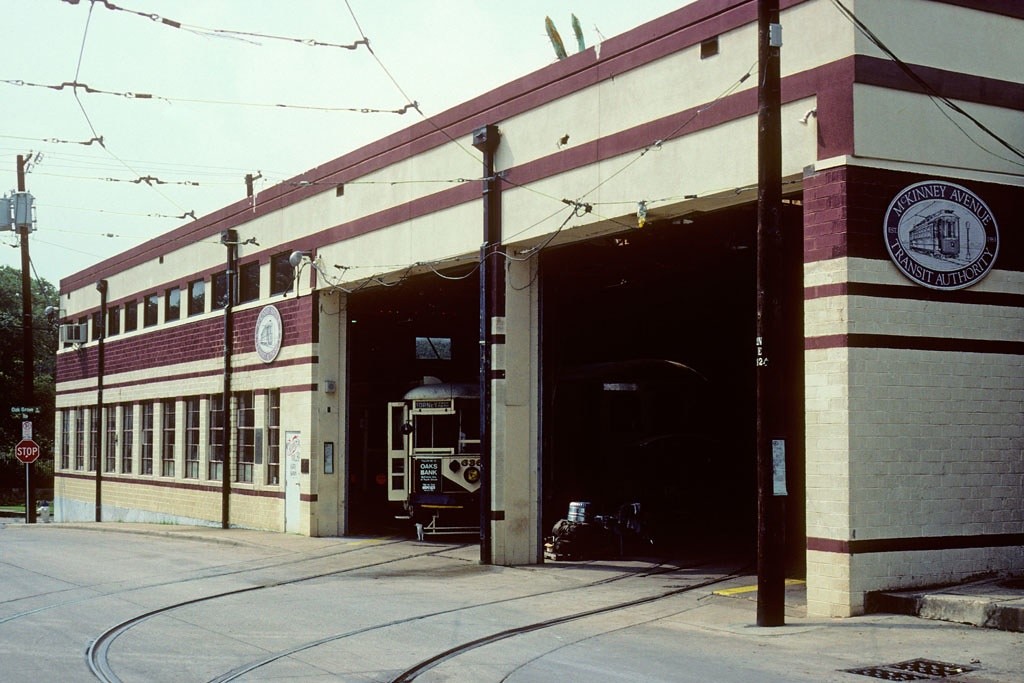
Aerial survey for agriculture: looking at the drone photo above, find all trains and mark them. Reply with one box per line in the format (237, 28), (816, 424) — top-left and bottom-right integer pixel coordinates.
(386, 383), (481, 544)
(908, 208), (961, 259)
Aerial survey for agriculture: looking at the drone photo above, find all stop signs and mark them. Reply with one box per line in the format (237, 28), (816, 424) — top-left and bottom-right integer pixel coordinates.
(15, 440), (40, 464)
